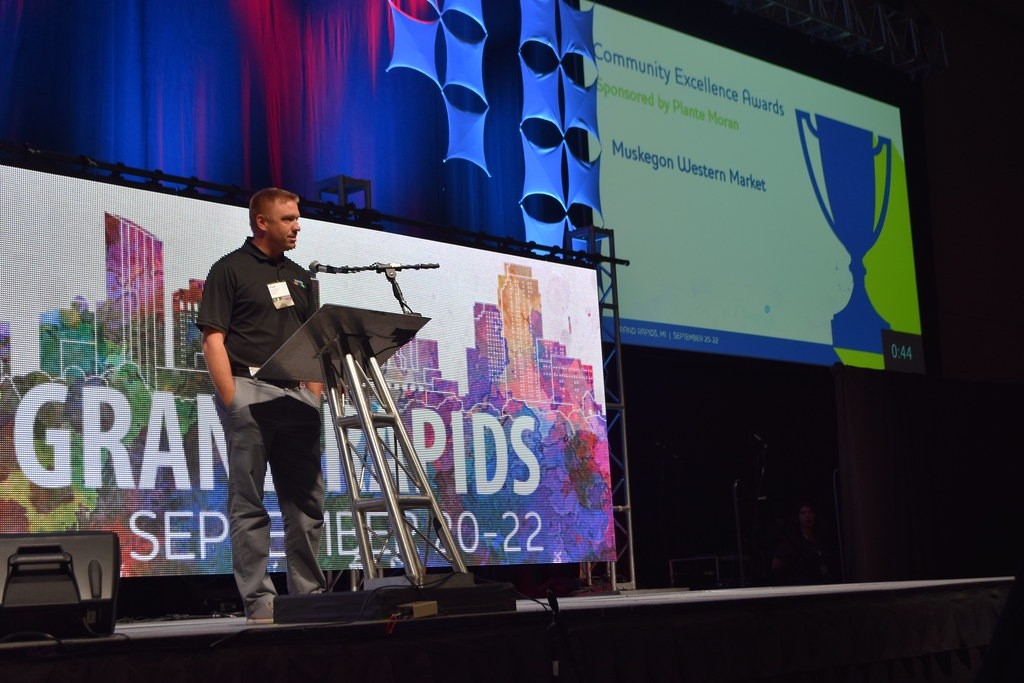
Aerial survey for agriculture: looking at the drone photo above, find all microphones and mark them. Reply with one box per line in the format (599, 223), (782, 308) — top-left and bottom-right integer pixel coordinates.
(309, 261), (337, 274)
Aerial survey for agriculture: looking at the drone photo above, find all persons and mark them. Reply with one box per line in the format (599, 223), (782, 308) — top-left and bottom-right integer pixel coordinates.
(194, 188), (326, 625)
(778, 503), (826, 583)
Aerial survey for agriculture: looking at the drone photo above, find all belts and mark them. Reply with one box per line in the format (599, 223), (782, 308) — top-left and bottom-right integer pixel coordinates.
(232, 366), (299, 389)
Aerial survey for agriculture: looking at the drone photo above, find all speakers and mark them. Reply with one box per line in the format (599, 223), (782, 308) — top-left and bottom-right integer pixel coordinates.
(0, 531), (122, 644)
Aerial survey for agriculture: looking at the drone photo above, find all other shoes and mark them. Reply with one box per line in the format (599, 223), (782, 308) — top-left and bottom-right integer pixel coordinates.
(246, 605), (274, 625)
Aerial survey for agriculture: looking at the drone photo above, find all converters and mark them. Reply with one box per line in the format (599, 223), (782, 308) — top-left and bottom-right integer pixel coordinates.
(396, 600), (438, 619)
